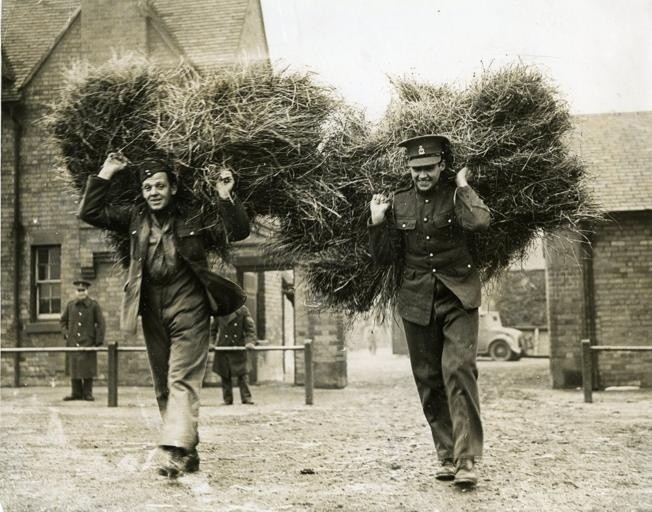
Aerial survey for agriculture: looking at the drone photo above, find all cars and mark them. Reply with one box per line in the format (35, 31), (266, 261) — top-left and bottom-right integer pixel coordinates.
(477, 313), (534, 362)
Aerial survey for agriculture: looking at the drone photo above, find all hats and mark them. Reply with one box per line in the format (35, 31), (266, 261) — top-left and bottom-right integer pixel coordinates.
(139, 162), (166, 184)
(73, 280), (91, 289)
(396, 135), (450, 168)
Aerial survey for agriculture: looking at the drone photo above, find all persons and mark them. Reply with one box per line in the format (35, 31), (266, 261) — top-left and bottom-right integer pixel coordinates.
(59, 281), (105, 401)
(367, 135), (491, 487)
(209, 305), (257, 405)
(76, 150), (251, 478)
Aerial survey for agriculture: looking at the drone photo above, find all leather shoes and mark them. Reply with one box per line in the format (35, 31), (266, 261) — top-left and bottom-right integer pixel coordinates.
(244, 401), (253, 404)
(64, 397), (94, 400)
(436, 460), (477, 488)
(157, 451), (200, 479)
